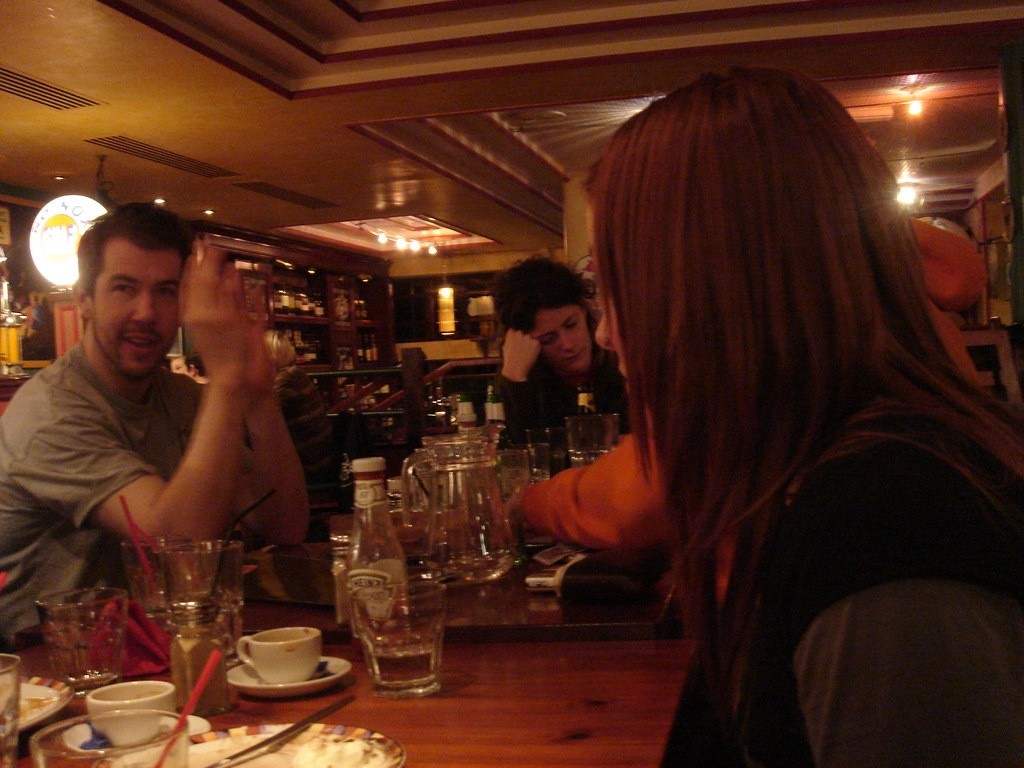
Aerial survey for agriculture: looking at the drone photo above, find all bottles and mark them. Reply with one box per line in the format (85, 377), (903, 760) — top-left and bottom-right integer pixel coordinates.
(480, 385), (517, 501)
(574, 383), (599, 417)
(456, 391), (476, 437)
(328, 455), (408, 649)
(163, 598), (234, 716)
(272, 284), (379, 366)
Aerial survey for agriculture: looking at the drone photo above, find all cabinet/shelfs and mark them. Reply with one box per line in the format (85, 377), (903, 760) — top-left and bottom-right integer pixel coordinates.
(176, 217), (401, 430)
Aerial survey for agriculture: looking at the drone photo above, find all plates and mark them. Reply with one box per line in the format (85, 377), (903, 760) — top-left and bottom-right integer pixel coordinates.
(92, 722), (407, 767)
(228, 655), (353, 696)
(1, 675), (75, 735)
(60, 715), (215, 756)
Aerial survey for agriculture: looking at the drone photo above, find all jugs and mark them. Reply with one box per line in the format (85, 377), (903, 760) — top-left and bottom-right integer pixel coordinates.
(405, 426), (519, 586)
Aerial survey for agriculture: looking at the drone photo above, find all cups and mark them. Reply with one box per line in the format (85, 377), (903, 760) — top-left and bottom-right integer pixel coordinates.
(237, 628), (325, 683)
(0, 652), (21, 768)
(30, 709), (190, 768)
(33, 587), (128, 699)
(161, 540), (247, 668)
(497, 413), (622, 551)
(120, 533), (191, 632)
(85, 681), (177, 746)
(353, 578), (451, 698)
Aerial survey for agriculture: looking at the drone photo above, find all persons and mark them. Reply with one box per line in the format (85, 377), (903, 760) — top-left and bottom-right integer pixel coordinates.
(496, 258), (630, 449)
(0, 201), (309, 649)
(501, 420), (670, 549)
(587, 67), (1024, 768)
(272, 329), (335, 482)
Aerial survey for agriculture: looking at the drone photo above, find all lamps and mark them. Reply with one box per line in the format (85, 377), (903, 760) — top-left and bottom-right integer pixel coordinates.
(437, 252), (459, 334)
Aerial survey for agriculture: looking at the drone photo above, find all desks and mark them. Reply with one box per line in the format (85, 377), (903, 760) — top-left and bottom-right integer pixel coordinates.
(0, 633), (709, 768)
(290, 533), (693, 638)
